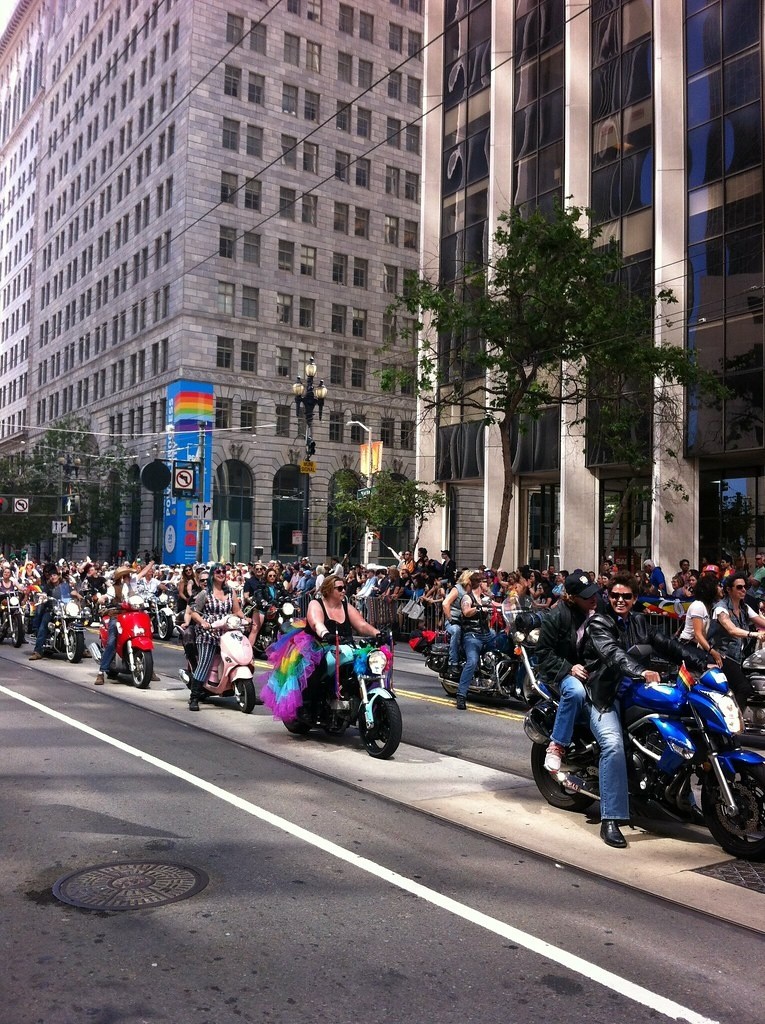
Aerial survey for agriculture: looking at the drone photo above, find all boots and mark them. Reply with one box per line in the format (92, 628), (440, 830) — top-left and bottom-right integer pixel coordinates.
(254, 697), (264, 703)
(187, 678), (205, 712)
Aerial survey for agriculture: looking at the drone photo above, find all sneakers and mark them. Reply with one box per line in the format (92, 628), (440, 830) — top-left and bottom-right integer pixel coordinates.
(544, 742), (563, 772)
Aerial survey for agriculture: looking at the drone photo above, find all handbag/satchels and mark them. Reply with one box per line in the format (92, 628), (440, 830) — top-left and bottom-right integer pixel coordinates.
(397, 599), (425, 620)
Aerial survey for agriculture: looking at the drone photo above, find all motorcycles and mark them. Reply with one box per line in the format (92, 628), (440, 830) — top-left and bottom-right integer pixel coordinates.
(281, 622), (403, 759)
(423, 609), (541, 706)
(248, 595), (301, 656)
(524, 646), (765, 870)
(0, 590), (95, 665)
(84, 586), (176, 641)
(175, 602), (268, 713)
(87, 593), (154, 689)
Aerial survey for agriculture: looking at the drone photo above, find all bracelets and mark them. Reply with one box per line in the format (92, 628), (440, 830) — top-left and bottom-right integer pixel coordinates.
(747, 631), (751, 637)
(320, 630), (328, 638)
(709, 648), (713, 653)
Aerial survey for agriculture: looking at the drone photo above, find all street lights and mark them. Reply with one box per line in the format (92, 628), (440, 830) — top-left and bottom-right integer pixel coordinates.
(58, 444), (82, 560)
(345, 420), (373, 566)
(294, 354), (329, 556)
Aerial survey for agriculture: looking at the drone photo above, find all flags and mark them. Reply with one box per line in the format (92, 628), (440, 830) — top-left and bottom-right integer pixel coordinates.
(677, 664), (695, 694)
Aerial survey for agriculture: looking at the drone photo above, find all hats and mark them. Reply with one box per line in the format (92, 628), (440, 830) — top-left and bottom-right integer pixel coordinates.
(196, 565), (206, 571)
(704, 565), (719, 573)
(305, 570), (311, 576)
(441, 550), (449, 555)
(114, 566), (137, 581)
(25, 560), (34, 569)
(565, 572), (601, 600)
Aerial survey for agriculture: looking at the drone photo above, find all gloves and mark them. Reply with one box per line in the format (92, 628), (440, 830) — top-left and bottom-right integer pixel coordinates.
(324, 632), (338, 645)
(381, 633), (397, 646)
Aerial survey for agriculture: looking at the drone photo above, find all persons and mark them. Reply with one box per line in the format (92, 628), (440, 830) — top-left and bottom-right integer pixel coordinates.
(0, 546), (765, 848)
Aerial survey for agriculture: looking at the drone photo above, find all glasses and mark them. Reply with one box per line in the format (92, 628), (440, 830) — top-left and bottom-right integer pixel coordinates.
(185, 568), (192, 572)
(609, 592), (632, 600)
(268, 574), (277, 578)
(333, 585), (347, 591)
(734, 584), (747, 589)
(200, 578), (208, 583)
(256, 567), (262, 571)
(215, 570), (226, 574)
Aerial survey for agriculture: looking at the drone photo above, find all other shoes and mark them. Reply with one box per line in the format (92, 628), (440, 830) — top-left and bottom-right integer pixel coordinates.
(94, 674), (106, 685)
(29, 652), (42, 661)
(150, 671), (161, 682)
(23, 636), (29, 644)
(451, 666), (460, 673)
(83, 650), (92, 657)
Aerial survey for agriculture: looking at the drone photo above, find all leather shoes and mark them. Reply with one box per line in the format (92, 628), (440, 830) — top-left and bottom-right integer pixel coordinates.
(599, 822), (628, 848)
(457, 696), (467, 710)
